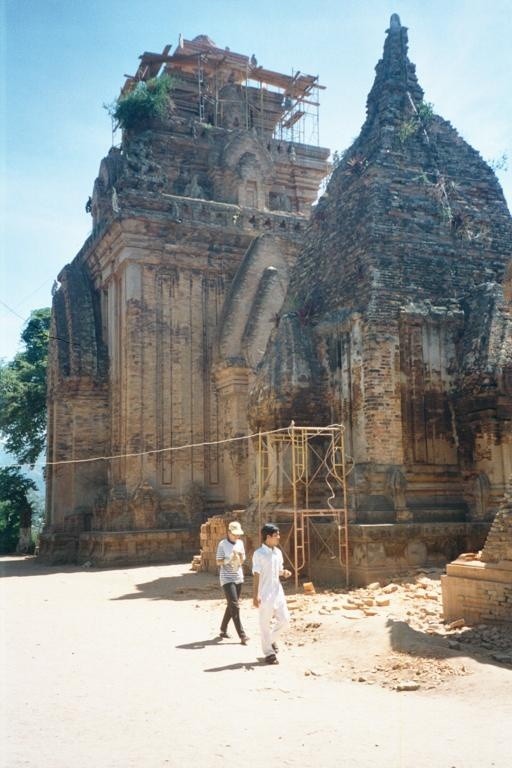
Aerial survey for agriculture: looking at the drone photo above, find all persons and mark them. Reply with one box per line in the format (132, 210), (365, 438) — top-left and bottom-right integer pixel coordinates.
(250, 522), (291, 667)
(214, 519), (253, 646)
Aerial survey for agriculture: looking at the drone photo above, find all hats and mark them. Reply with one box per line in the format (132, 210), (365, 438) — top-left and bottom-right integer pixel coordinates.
(259, 523), (275, 541)
(228, 521), (245, 536)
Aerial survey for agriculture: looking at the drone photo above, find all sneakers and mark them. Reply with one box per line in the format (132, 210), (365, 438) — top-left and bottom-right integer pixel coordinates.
(240, 637), (250, 644)
(220, 632), (231, 639)
(272, 642), (279, 654)
(265, 654), (278, 664)
